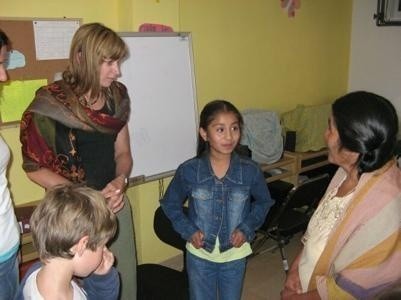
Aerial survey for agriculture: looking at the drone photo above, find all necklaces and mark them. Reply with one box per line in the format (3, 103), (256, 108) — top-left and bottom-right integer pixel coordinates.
(91, 91), (100, 106)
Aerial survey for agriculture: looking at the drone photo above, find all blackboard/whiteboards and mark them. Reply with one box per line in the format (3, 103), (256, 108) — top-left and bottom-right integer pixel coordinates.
(116, 33), (200, 187)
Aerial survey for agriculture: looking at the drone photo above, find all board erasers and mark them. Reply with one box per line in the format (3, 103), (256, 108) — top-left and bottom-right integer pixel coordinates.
(128, 175), (145, 186)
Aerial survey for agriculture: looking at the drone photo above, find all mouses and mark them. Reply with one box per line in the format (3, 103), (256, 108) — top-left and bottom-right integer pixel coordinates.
(273, 168), (282, 175)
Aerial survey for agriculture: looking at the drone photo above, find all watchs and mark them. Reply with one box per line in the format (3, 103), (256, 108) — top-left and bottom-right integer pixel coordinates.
(118, 174), (129, 187)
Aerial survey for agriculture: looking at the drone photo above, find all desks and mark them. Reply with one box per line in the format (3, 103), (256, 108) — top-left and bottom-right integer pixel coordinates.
(284, 151), (330, 185)
(260, 152), (299, 186)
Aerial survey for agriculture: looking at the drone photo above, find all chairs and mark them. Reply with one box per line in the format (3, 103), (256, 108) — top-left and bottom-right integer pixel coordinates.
(137, 207), (188, 300)
(252, 173), (330, 275)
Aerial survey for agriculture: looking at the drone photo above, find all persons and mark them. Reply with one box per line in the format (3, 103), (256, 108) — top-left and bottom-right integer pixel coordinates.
(161, 101), (272, 300)
(20, 22), (137, 299)
(0, 28), (22, 300)
(280, 91), (401, 300)
(13, 182), (121, 300)
